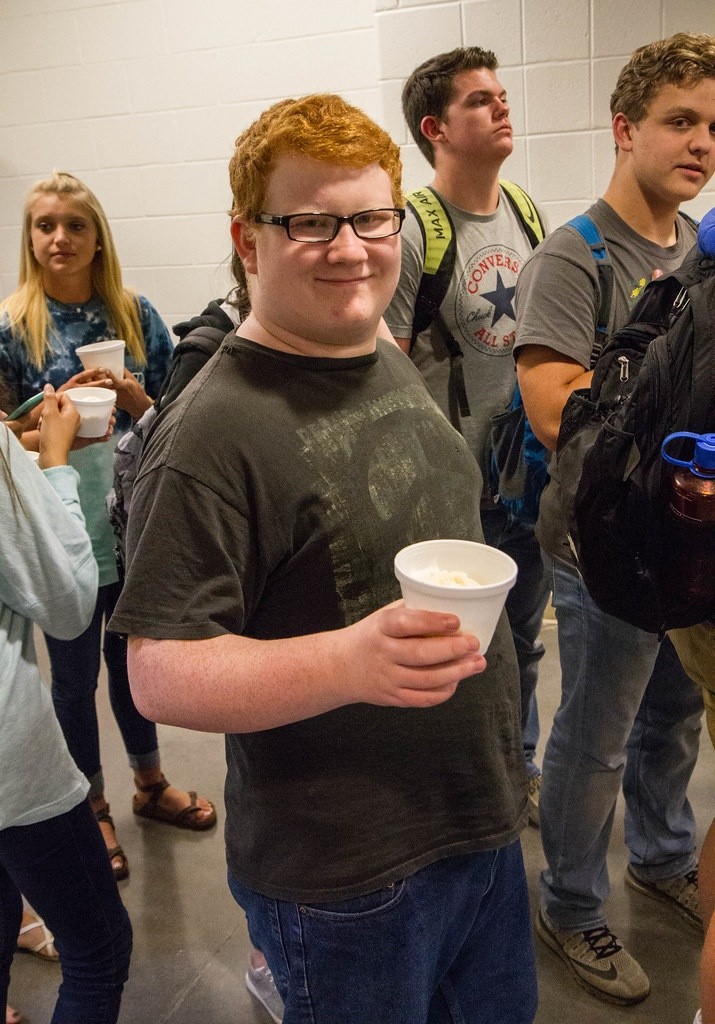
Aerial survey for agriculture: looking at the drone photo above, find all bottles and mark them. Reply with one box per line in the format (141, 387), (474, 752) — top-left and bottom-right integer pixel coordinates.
(659, 429), (715, 526)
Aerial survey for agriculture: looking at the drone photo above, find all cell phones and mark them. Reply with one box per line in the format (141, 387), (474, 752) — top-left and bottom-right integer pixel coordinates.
(4, 391), (45, 422)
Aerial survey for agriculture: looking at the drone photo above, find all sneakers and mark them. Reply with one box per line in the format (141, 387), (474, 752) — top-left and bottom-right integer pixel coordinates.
(535, 905), (650, 1005)
(625, 860), (702, 930)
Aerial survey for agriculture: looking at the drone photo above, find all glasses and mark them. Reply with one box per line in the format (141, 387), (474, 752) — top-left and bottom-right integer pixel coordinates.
(257, 208), (406, 243)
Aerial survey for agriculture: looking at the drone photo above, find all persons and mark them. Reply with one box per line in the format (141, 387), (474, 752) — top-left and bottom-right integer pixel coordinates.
(104, 92), (540, 1024)
(163, 35), (715, 1024)
(0, 174), (216, 1024)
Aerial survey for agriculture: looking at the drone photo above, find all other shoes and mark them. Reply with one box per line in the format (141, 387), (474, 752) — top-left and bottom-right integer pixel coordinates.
(247, 947), (285, 1024)
(528, 773), (542, 827)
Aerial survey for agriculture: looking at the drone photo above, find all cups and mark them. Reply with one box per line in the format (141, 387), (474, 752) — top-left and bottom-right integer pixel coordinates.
(76, 338), (126, 384)
(392, 540), (519, 657)
(62, 387), (116, 438)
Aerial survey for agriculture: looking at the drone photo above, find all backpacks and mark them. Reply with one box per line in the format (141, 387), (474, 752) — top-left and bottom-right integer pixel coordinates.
(104, 327), (227, 588)
(485, 215), (613, 527)
(557, 246), (715, 642)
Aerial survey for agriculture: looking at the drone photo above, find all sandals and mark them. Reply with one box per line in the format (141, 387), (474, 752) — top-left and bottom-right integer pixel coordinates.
(16, 909), (60, 961)
(132, 773), (216, 830)
(95, 803), (128, 879)
